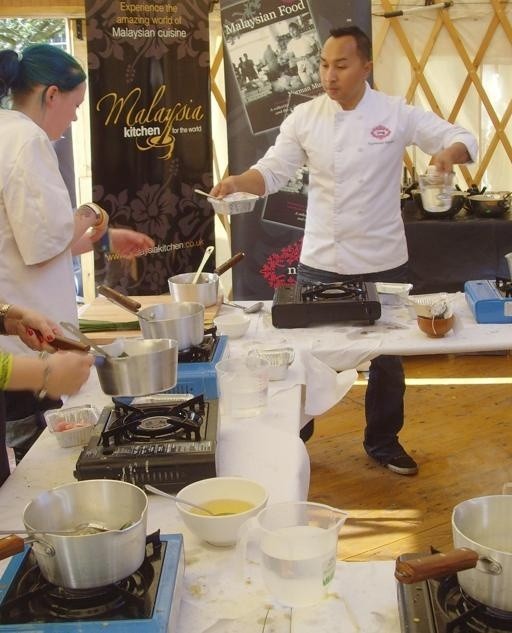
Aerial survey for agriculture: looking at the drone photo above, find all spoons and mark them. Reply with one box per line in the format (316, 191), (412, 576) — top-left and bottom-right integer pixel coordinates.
(60, 321), (114, 358)
(194, 188), (218, 199)
(224, 302), (264, 314)
(0, 521), (112, 535)
(192, 245), (214, 284)
(144, 483), (236, 517)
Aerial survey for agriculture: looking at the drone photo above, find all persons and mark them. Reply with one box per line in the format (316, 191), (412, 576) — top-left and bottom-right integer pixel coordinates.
(283, 22), (321, 85)
(262, 43), (283, 74)
(244, 52), (259, 81)
(208, 25), (479, 475)
(0, 296), (96, 486)
(238, 56), (253, 81)
(0, 42), (156, 466)
(233, 62), (240, 80)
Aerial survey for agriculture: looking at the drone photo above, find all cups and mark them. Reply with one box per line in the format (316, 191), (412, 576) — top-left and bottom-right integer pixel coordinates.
(237, 501), (349, 609)
(215, 355), (270, 420)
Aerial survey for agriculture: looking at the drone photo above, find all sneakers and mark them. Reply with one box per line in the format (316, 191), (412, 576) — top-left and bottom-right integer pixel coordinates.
(386, 451), (417, 475)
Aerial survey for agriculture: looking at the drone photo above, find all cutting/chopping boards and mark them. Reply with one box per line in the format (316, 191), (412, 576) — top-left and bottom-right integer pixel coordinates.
(80, 295), (224, 344)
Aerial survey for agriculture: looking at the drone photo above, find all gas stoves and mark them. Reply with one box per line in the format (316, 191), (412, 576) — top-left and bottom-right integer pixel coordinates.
(1, 530), (186, 633)
(160, 325), (229, 401)
(395, 546), (512, 633)
(271, 277), (381, 330)
(73, 393), (220, 495)
(464, 278), (512, 323)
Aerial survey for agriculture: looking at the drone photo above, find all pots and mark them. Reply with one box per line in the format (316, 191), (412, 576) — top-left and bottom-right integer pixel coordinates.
(97, 284), (205, 351)
(168, 250), (246, 308)
(394, 482), (512, 612)
(0, 480), (149, 590)
(29, 324), (179, 397)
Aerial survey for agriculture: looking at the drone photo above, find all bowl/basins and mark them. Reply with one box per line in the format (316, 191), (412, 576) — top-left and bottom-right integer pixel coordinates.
(215, 312), (250, 339)
(469, 194), (510, 218)
(207, 192), (260, 215)
(44, 404), (98, 448)
(247, 348), (294, 381)
(419, 313), (455, 339)
(375, 281), (412, 306)
(411, 190), (470, 219)
(406, 292), (446, 319)
(175, 476), (268, 548)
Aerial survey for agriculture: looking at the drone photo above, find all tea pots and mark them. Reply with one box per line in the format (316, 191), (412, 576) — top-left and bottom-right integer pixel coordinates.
(418, 171), (456, 213)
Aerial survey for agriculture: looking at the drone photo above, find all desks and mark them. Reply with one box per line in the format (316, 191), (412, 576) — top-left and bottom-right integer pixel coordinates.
(0, 516), (412, 633)
(1, 357), (309, 557)
(76, 285), (511, 372)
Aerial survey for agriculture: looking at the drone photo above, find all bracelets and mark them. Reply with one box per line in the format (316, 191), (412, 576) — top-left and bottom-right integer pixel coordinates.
(81, 203), (102, 226)
(93, 229), (110, 255)
(92, 199), (107, 231)
(1, 353), (13, 393)
(32, 351), (52, 399)
(0, 301), (15, 337)
(0, 301), (11, 337)
